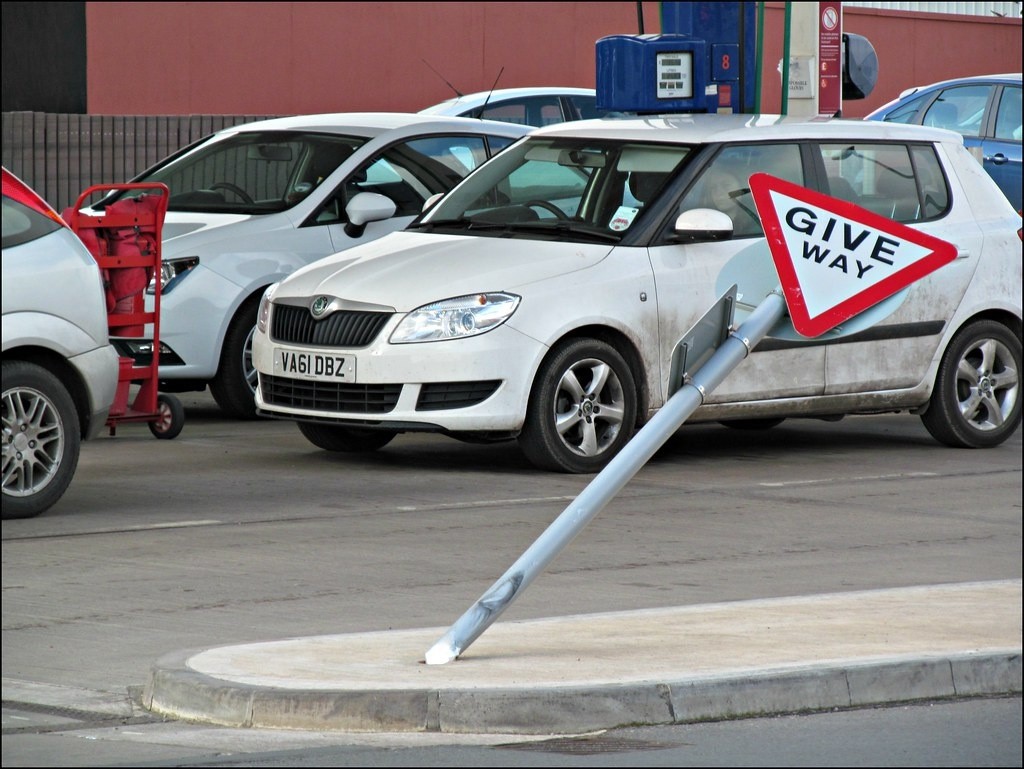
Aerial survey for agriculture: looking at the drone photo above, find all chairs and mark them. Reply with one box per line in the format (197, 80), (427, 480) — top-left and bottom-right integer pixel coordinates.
(311, 142), (367, 222)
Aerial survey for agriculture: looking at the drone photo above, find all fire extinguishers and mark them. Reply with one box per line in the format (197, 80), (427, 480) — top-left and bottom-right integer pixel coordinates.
(107, 193), (146, 337)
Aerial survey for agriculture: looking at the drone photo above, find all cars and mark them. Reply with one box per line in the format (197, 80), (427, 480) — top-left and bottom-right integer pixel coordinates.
(835, 72), (1023, 216)
(350, 85), (634, 207)
(68, 106), (645, 422)
(245, 110), (1023, 476)
(0, 164), (121, 520)
